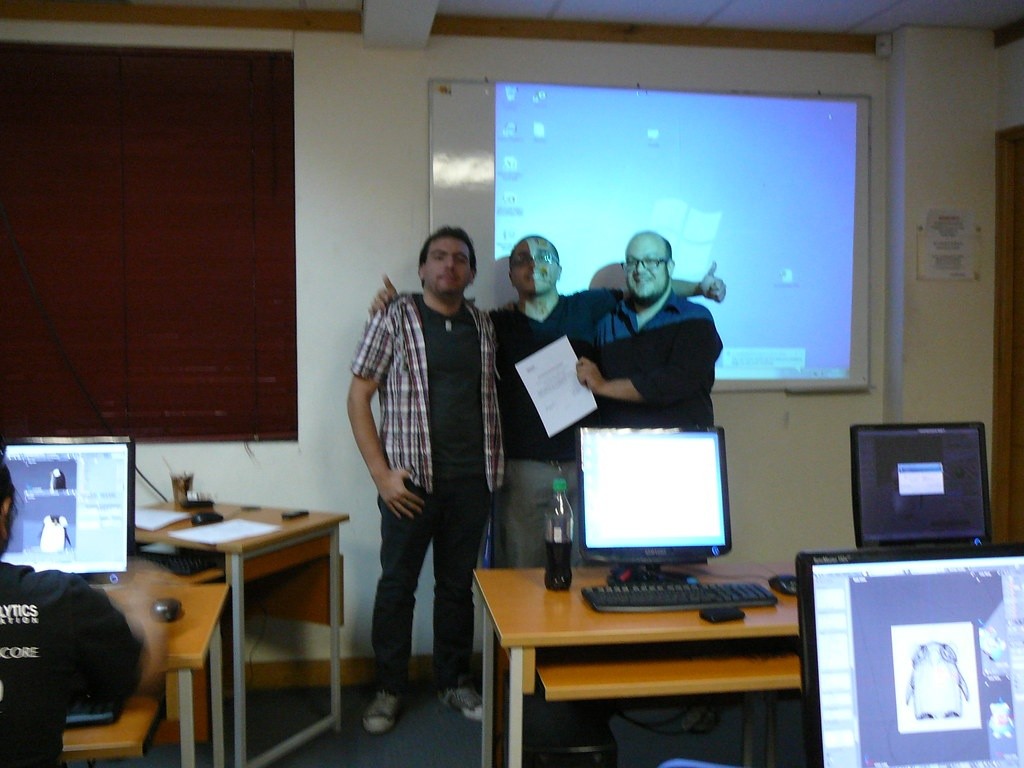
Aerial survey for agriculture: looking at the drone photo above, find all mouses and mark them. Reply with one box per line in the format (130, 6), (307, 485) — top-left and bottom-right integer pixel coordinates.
(768, 574), (798, 596)
(150, 598), (180, 624)
(191, 512), (224, 525)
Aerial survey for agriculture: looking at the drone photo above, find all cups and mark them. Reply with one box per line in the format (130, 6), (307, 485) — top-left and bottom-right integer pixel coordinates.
(170, 473), (193, 512)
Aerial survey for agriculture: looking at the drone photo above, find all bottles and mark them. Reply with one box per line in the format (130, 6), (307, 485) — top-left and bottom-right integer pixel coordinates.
(543, 478), (575, 591)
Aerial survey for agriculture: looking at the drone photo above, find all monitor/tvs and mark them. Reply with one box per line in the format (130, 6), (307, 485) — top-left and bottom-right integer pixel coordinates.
(0, 437), (136, 580)
(795, 545), (1024, 768)
(575, 423), (733, 585)
(849, 423), (991, 550)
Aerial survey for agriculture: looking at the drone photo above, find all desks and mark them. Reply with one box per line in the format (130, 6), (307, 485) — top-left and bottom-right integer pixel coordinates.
(473, 560), (801, 768)
(105, 582), (232, 768)
(59, 697), (159, 768)
(133, 501), (350, 768)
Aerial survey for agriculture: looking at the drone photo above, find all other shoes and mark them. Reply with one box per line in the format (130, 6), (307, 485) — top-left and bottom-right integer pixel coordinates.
(680, 702), (718, 733)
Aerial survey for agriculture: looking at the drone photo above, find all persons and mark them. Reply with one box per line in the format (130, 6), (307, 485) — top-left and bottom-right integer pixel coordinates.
(576, 231), (724, 431)
(347, 226), (506, 736)
(0, 451), (146, 768)
(368, 234), (729, 708)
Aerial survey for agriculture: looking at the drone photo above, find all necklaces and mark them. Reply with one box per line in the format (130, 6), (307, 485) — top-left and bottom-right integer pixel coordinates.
(418, 292), (465, 332)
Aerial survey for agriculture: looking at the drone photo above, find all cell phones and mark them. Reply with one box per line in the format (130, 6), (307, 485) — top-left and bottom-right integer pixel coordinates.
(281, 510), (309, 519)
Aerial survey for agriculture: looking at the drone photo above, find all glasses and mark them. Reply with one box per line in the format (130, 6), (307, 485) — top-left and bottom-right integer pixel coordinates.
(511, 250), (561, 272)
(621, 254), (669, 272)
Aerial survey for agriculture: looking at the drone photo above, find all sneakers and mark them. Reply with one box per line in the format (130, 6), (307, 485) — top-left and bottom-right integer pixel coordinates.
(441, 687), (483, 720)
(362, 690), (399, 736)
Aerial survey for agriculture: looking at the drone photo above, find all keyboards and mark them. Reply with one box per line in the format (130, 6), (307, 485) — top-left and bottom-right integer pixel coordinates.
(582, 583), (778, 612)
(134, 549), (216, 576)
(63, 700), (123, 727)
